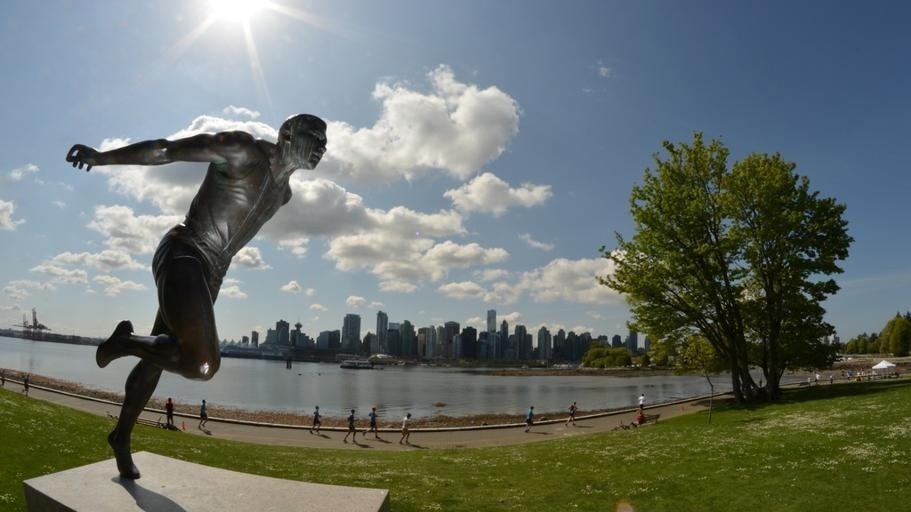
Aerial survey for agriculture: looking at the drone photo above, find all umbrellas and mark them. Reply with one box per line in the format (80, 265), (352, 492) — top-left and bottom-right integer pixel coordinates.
(872, 360), (896, 375)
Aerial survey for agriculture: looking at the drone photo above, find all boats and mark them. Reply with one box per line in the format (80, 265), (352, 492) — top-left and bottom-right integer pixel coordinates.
(338, 359), (375, 369)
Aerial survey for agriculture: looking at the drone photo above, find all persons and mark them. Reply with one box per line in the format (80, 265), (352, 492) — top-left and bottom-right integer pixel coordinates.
(636, 406), (644, 423)
(363, 408), (381, 439)
(525, 406), (535, 432)
(566, 402), (577, 426)
(343, 409), (356, 444)
(638, 393), (646, 410)
(310, 405), (322, 434)
(165, 398), (176, 425)
(22, 374), (30, 397)
(66, 113), (327, 478)
(814, 367), (900, 384)
(399, 413), (412, 445)
(1, 370), (6, 386)
(198, 399), (208, 429)
